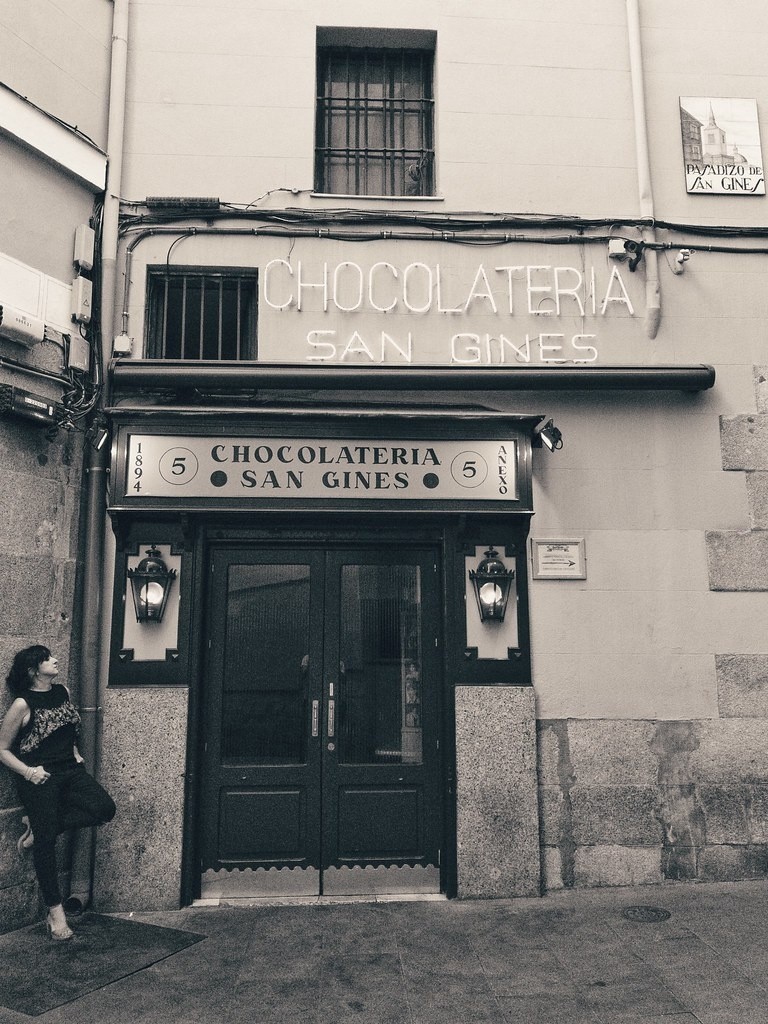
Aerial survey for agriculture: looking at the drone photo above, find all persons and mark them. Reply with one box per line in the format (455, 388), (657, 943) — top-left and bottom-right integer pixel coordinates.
(0, 644), (117, 941)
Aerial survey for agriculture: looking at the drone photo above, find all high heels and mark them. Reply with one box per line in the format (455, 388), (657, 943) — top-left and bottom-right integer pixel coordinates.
(46, 914), (73, 940)
(17, 816), (35, 859)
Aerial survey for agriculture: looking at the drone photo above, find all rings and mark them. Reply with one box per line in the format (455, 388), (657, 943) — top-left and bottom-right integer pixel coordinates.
(41, 777), (48, 784)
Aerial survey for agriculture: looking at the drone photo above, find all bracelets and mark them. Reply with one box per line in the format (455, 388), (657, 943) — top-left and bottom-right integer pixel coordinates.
(24, 766), (36, 780)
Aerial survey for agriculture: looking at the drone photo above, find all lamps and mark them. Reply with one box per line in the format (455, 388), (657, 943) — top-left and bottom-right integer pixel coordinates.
(468, 545), (515, 623)
(532, 417), (563, 454)
(84, 418), (110, 451)
(127, 545), (178, 624)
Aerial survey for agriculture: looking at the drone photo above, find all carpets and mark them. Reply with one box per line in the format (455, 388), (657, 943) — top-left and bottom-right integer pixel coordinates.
(0, 913), (209, 1017)
